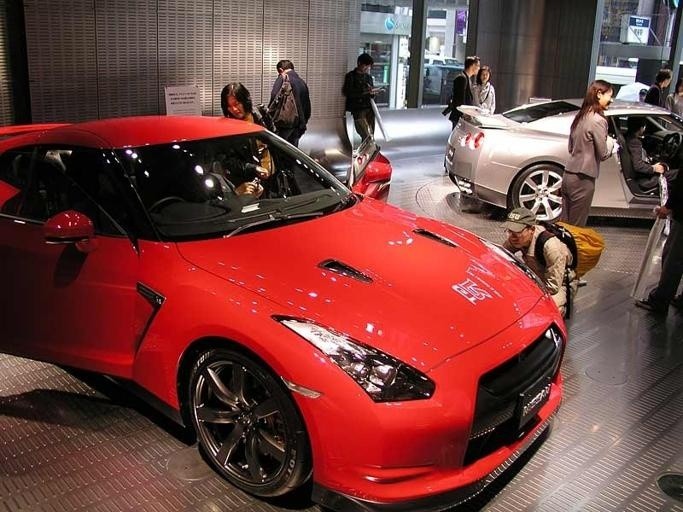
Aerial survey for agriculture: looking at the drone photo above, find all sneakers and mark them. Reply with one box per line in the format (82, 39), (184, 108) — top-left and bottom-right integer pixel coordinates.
(636, 300), (669, 316)
(671, 295), (683, 309)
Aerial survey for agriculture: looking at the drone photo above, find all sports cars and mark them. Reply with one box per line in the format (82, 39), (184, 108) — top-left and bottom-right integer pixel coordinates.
(444, 99), (683, 225)
(0, 115), (566, 512)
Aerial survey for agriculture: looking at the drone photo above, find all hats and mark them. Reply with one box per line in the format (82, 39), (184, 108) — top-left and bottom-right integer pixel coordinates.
(500, 207), (539, 232)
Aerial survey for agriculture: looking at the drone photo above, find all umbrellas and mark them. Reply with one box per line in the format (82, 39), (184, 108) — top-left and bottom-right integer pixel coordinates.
(658, 162), (671, 238)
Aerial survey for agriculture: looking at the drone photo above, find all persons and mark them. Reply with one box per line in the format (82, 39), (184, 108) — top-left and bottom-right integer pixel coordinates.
(471, 64), (496, 115)
(635, 155), (683, 317)
(559, 80), (614, 229)
(665, 77), (683, 118)
(449, 55), (480, 132)
(220, 82), (282, 199)
(645, 68), (673, 132)
(625, 117), (679, 189)
(341, 53), (376, 141)
(268, 59), (311, 196)
(210, 172), (264, 199)
(499, 207), (579, 321)
(639, 88), (648, 103)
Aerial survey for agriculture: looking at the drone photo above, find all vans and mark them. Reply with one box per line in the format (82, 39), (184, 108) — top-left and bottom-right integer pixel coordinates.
(404, 55), (459, 80)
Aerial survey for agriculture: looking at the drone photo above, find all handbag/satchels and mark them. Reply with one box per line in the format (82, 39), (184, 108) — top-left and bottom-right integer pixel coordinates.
(253, 105), (291, 195)
(269, 74), (298, 127)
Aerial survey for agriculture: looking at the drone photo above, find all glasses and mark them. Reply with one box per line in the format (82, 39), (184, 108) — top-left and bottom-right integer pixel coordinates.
(504, 229), (527, 237)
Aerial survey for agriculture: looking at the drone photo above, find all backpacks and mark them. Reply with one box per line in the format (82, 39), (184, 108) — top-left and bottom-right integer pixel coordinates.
(256, 104), (301, 199)
(534, 219), (605, 318)
(344, 71), (353, 120)
